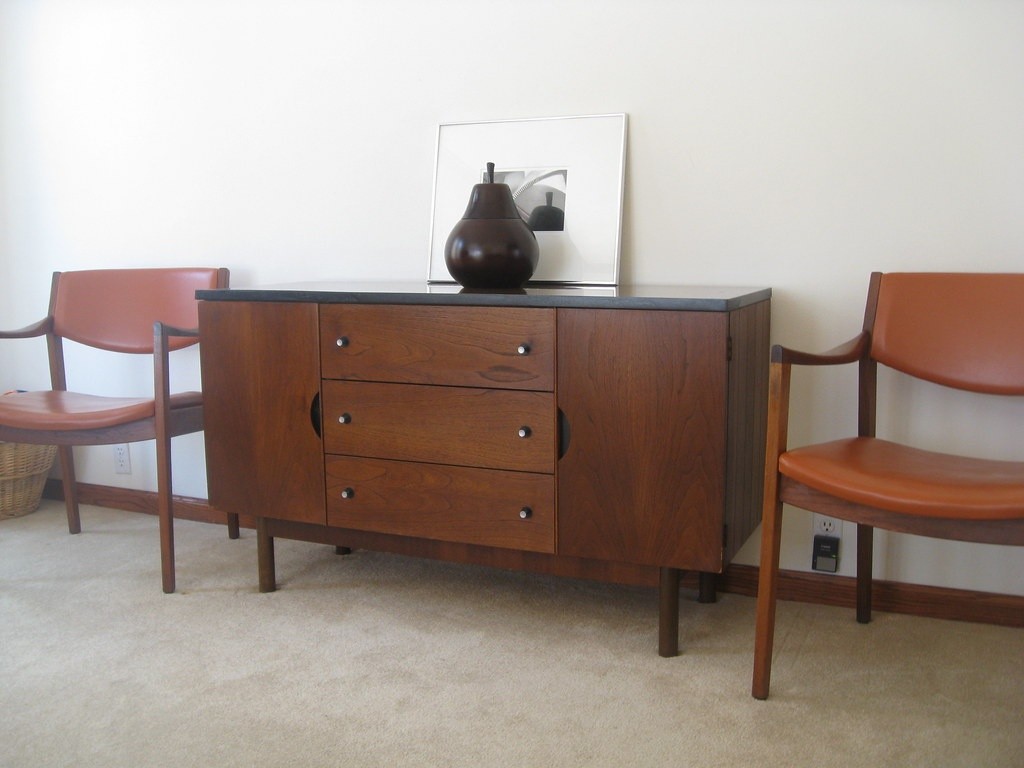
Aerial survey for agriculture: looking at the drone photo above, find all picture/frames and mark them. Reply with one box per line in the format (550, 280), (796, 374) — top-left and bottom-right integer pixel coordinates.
(427, 113), (627, 289)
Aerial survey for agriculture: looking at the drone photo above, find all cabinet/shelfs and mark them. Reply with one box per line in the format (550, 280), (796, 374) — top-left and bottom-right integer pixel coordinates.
(193, 281), (777, 661)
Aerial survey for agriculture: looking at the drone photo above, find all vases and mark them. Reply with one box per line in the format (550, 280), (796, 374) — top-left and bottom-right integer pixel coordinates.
(443, 183), (539, 292)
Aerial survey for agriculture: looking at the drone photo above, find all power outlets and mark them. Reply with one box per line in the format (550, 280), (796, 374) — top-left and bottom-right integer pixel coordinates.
(112, 443), (131, 474)
(812, 512), (841, 546)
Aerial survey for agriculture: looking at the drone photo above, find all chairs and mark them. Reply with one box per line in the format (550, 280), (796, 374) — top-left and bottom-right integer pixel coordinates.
(0, 265), (238, 596)
(751, 270), (1024, 708)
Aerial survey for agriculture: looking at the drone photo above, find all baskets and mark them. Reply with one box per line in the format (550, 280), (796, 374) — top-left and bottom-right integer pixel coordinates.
(0, 390), (59, 520)
